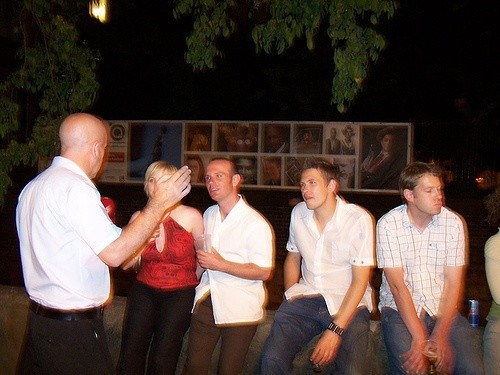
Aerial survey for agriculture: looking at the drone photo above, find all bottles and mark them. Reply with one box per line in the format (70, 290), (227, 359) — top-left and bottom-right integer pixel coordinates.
(468, 300), (480, 328)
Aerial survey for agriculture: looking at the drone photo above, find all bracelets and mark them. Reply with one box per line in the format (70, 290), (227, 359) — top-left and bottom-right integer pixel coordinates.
(326, 322), (345, 337)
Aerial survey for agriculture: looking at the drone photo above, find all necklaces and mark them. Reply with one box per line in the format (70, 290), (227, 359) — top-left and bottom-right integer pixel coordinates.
(159, 209), (174, 223)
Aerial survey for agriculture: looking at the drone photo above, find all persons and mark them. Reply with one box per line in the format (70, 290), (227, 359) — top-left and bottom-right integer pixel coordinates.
(15, 113), (191, 375)
(126, 120), (407, 192)
(113, 161), (203, 375)
(177, 158), (275, 374)
(483, 189), (500, 375)
(256, 155), (375, 375)
(375, 159), (485, 375)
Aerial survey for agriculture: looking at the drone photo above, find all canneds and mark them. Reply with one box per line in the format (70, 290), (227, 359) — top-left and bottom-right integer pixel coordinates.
(468, 300), (479, 328)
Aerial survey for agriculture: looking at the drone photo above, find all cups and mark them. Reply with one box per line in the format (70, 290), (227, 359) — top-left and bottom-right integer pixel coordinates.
(194, 235), (212, 255)
